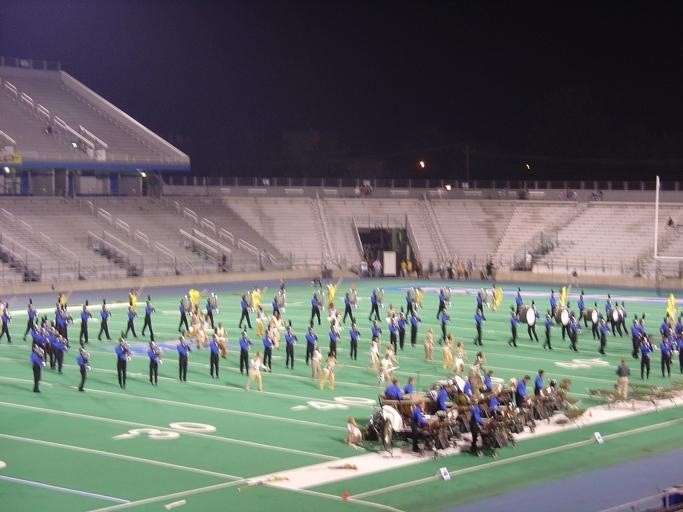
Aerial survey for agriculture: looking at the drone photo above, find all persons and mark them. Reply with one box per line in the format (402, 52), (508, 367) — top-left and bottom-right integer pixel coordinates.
(344, 392), (543, 453)
(354, 184), (372, 195)
(359, 258), (493, 279)
(525, 253), (533, 271)
(614, 358), (629, 399)
(664, 213), (675, 231)
(562, 187), (606, 199)
(568, 267), (580, 287)
(0, 276), (682, 392)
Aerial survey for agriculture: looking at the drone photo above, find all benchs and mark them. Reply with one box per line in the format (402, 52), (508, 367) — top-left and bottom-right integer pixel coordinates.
(0, 75), (187, 163)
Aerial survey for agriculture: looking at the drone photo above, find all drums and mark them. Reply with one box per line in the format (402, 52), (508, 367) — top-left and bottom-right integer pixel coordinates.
(555, 309), (569, 326)
(519, 306), (535, 326)
(586, 308), (598, 323)
(607, 308), (618, 322)
